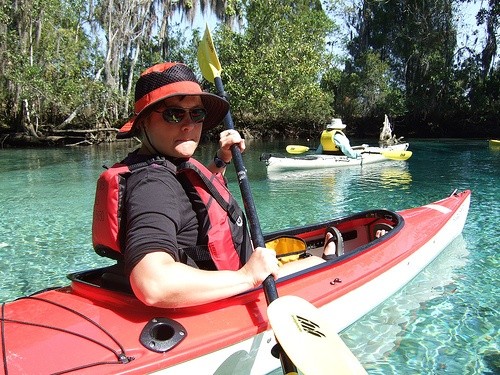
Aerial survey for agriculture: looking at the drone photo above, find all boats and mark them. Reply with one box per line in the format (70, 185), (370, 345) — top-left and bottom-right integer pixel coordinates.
(489, 139), (500, 147)
(265, 142), (410, 172)
(0, 188), (472, 375)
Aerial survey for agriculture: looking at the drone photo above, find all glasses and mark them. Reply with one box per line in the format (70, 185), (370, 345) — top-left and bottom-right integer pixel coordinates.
(154, 106), (209, 123)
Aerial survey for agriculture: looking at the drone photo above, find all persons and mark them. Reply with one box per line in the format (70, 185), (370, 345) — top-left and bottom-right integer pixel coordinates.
(315, 118), (362, 160)
(116, 62), (394, 309)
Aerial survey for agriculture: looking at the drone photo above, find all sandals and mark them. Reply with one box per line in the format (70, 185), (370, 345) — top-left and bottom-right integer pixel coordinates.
(322, 226), (344, 262)
(373, 223), (394, 240)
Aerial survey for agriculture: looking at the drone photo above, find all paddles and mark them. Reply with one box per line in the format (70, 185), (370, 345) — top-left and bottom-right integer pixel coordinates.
(197, 20), (369, 375)
(286, 145), (413, 160)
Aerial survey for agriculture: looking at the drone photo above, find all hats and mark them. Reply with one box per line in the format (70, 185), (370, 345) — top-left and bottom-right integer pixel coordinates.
(116, 63), (230, 139)
(327, 119), (346, 129)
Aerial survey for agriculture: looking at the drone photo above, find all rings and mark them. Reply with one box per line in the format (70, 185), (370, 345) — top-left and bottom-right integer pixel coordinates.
(225, 132), (231, 136)
